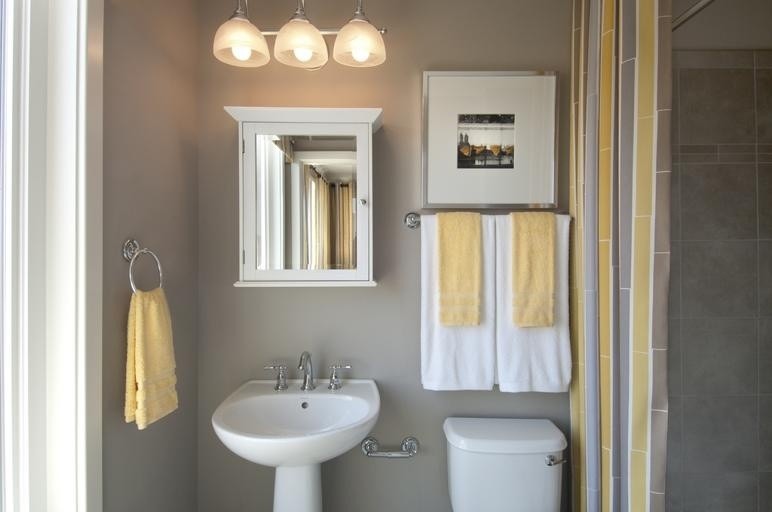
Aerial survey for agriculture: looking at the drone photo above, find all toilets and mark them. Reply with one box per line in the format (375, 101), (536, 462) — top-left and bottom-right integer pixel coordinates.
(442, 415), (568, 511)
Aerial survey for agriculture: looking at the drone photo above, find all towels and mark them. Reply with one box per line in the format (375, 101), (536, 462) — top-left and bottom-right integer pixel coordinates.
(509, 210), (558, 329)
(419, 212), (496, 395)
(435, 211), (485, 328)
(123, 287), (181, 433)
(496, 212), (574, 395)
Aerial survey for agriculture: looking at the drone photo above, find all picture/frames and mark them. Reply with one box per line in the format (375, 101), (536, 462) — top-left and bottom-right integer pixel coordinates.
(420, 69), (563, 212)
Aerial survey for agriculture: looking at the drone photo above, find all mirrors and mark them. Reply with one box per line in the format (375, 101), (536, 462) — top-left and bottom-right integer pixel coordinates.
(223, 104), (386, 290)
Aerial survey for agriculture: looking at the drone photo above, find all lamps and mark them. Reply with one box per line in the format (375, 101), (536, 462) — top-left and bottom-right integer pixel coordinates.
(211, 0), (389, 70)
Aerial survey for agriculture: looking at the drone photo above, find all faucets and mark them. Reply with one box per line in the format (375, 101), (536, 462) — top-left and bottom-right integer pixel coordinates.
(297, 350), (314, 391)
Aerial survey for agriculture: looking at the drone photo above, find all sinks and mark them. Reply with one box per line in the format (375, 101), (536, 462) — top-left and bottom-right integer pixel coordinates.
(212, 377), (382, 466)
